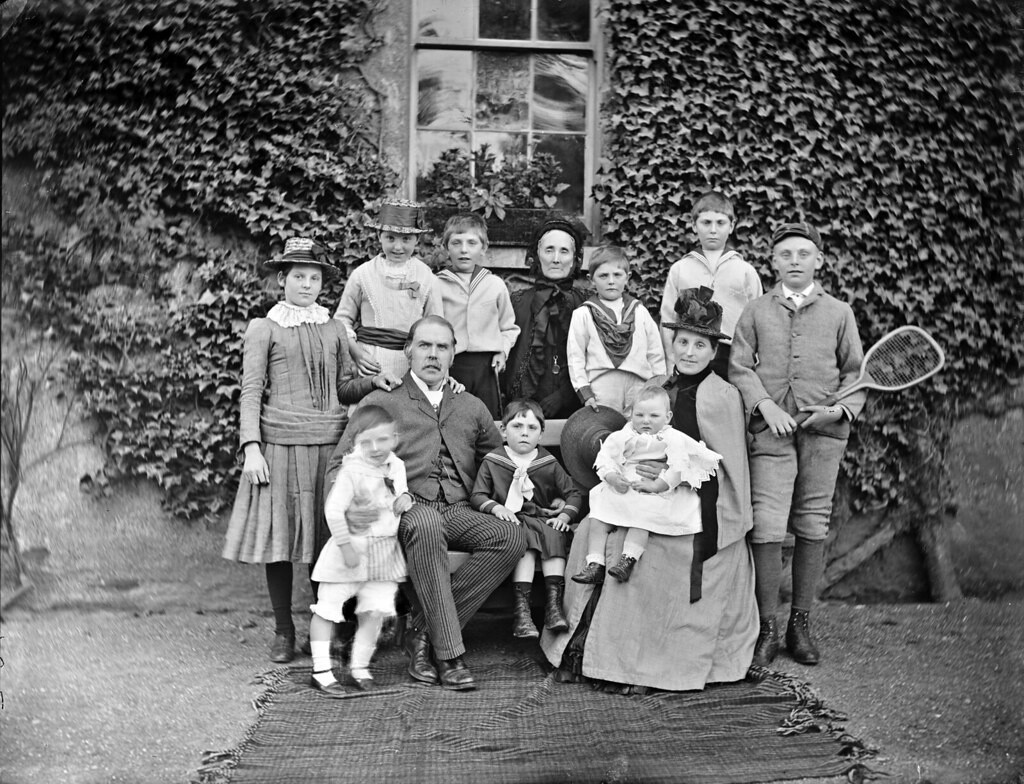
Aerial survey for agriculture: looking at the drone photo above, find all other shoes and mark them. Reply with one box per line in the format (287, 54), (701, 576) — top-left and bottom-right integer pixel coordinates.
(348, 672), (378, 693)
(609, 555), (636, 582)
(270, 633), (294, 661)
(571, 562), (605, 586)
(312, 669), (346, 696)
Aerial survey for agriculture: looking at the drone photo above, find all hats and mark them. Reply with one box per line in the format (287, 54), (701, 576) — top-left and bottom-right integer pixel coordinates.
(560, 404), (629, 488)
(662, 287), (731, 340)
(365, 201), (434, 233)
(262, 237), (340, 276)
(773, 223), (820, 251)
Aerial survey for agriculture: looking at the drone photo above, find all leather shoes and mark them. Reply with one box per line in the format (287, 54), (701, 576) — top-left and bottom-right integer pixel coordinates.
(402, 627), (437, 682)
(435, 654), (476, 691)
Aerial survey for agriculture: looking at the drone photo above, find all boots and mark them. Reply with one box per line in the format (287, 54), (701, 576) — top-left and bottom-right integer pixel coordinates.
(786, 608), (819, 663)
(544, 584), (570, 631)
(755, 616), (780, 665)
(513, 584), (540, 639)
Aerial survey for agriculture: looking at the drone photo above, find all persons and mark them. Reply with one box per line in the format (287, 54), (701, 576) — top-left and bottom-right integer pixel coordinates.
(660, 193), (763, 374)
(223, 237), (403, 662)
(471, 400), (582, 636)
(309, 404), (415, 696)
(566, 246), (667, 415)
(571, 385), (723, 584)
(321, 315), (523, 689)
(729, 223), (869, 664)
(435, 215), (520, 422)
(330, 202), (466, 417)
(539, 286), (760, 696)
(499, 223), (592, 417)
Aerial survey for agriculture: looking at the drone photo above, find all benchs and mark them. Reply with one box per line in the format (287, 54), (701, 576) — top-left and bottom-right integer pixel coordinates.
(397, 420), (569, 645)
